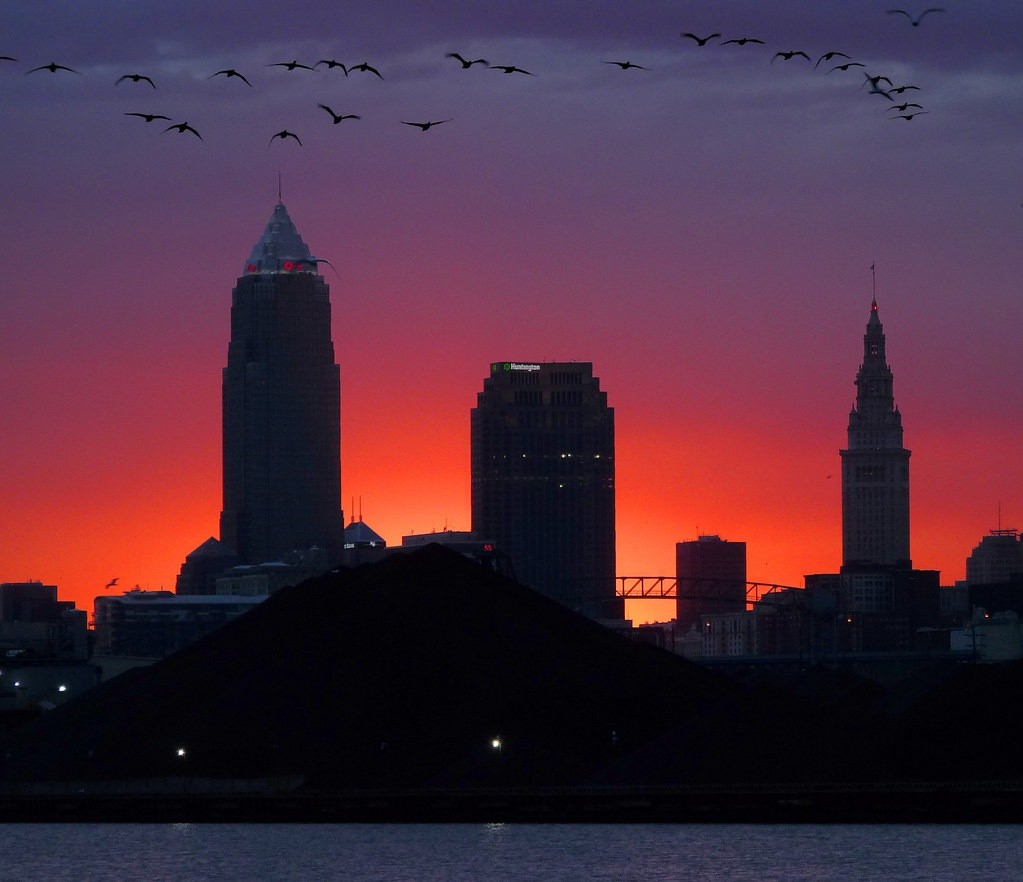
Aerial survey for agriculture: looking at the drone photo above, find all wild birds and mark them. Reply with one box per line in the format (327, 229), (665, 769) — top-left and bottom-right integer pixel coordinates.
(125, 113), (203, 141)
(886, 8), (944, 26)
(295, 257), (338, 276)
(270, 60), (383, 80)
(0, 56), (16, 61)
(447, 52), (534, 78)
(105, 578), (120, 590)
(682, 32), (720, 46)
(269, 130), (302, 146)
(605, 61), (645, 70)
(208, 69), (252, 87)
(719, 38), (764, 45)
(28, 62), (75, 72)
(319, 104), (359, 124)
(771, 51), (865, 76)
(115, 74), (156, 89)
(862, 71), (928, 121)
(402, 118), (451, 131)
